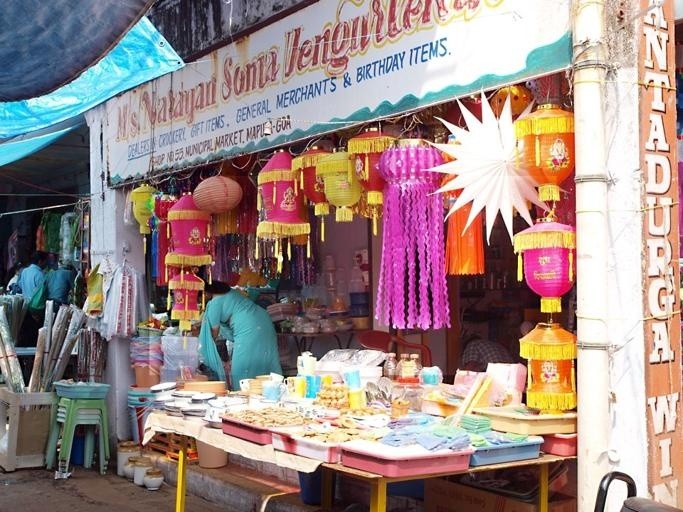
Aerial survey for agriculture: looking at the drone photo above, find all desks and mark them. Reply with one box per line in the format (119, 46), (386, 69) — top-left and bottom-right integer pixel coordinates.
(142, 413), (577, 512)
(276, 330), (356, 355)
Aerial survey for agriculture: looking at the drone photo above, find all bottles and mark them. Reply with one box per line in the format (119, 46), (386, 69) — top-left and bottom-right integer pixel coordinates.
(383, 353), (397, 380)
(410, 353), (423, 382)
(395, 354), (409, 382)
(325, 255), (366, 310)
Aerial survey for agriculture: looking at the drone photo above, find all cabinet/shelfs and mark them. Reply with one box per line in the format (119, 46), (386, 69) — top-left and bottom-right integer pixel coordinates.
(444, 212), (568, 375)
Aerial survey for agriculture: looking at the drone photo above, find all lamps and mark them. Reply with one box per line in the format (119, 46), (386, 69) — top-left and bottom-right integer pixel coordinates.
(263, 117), (272, 134)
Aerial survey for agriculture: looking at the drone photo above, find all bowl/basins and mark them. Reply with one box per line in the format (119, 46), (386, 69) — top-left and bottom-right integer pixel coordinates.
(150, 382), (217, 405)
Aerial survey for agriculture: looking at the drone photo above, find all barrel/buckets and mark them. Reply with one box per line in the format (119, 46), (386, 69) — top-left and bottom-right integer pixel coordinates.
(127, 386), (154, 445)
(130, 336), (162, 387)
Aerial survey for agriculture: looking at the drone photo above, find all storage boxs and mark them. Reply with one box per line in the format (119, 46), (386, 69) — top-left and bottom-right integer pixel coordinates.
(129, 336), (164, 387)
(423, 478), (577, 512)
(267, 303), (296, 322)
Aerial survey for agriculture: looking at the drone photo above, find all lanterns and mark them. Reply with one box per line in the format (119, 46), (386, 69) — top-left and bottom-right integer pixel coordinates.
(130, 125), (491, 350)
(490, 85), (577, 415)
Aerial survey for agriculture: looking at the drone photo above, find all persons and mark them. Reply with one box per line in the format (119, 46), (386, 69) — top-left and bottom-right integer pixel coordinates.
(4, 250), (79, 344)
(461, 335), (514, 373)
(197, 281), (283, 392)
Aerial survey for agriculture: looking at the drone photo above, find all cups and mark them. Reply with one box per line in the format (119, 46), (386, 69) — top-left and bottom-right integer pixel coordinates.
(344, 370), (363, 410)
(239, 373), (320, 400)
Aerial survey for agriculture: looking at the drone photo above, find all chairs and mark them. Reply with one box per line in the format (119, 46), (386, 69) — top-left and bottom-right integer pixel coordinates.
(360, 330), (432, 367)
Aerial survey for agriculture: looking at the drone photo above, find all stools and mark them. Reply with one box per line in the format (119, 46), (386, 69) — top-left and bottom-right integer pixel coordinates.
(44, 397), (110, 476)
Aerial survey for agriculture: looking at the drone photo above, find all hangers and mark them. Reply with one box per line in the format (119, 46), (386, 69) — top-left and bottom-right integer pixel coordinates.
(93, 247), (131, 265)
